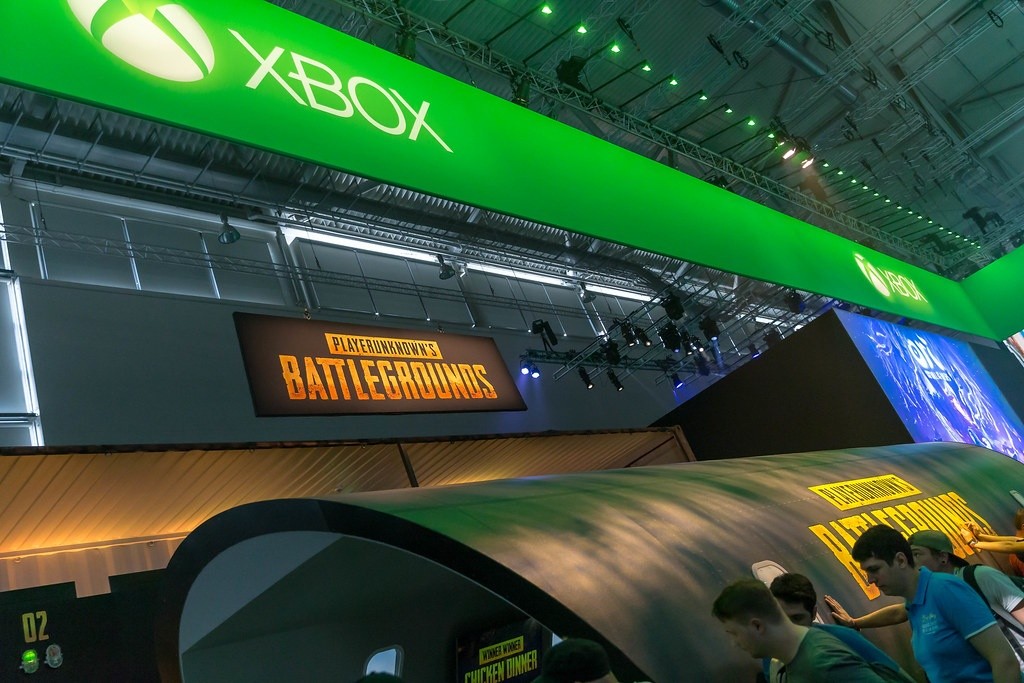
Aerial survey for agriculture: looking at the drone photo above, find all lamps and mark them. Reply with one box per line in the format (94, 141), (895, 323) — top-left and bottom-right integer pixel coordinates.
(581, 289), (596, 305)
(622, 326), (635, 347)
(531, 367), (540, 378)
(665, 297), (684, 321)
(748, 344), (760, 359)
(436, 254), (455, 280)
(703, 325), (721, 342)
(578, 368), (594, 390)
(672, 374), (683, 389)
(789, 295), (806, 314)
(521, 364), (530, 376)
(681, 332), (693, 356)
(772, 130), (814, 169)
(218, 215), (240, 244)
(544, 321), (558, 346)
(532, 324), (544, 335)
(608, 372), (624, 392)
(661, 324), (681, 353)
(634, 328), (652, 347)
(691, 336), (704, 353)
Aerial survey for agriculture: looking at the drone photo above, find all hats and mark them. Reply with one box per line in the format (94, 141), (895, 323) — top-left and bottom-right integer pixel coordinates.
(530, 638), (610, 683)
(907, 530), (969, 567)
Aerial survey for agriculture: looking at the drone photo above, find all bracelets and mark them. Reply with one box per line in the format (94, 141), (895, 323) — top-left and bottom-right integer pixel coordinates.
(852, 620), (860, 631)
(968, 538), (978, 546)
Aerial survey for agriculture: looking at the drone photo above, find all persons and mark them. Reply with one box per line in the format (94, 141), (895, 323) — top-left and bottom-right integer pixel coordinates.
(713, 507), (1024, 683)
(852, 524), (1021, 683)
